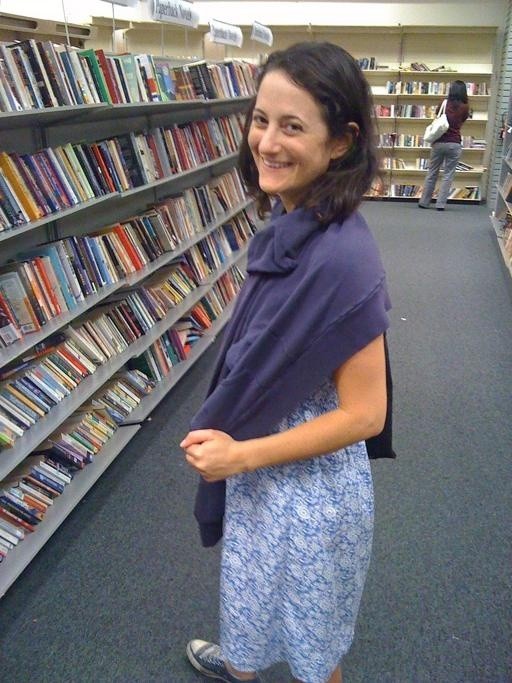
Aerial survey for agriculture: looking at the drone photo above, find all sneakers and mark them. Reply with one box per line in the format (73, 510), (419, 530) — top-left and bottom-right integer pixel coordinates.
(187, 639), (261, 683)
(419, 204), (428, 209)
(438, 208), (443, 211)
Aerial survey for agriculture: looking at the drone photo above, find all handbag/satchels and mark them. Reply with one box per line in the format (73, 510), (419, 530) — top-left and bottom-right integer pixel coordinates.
(423, 115), (449, 142)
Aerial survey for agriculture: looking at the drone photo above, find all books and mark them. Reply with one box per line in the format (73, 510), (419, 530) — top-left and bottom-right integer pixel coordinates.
(496, 139), (512, 268)
(357, 53), (489, 199)
(1, 35), (273, 564)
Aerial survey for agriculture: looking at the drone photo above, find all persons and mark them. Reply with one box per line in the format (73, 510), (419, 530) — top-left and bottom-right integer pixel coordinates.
(417, 78), (471, 212)
(178, 39), (401, 681)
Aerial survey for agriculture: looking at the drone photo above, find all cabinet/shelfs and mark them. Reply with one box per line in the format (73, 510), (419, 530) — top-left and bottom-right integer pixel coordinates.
(489, 117), (512, 278)
(362, 70), (493, 201)
